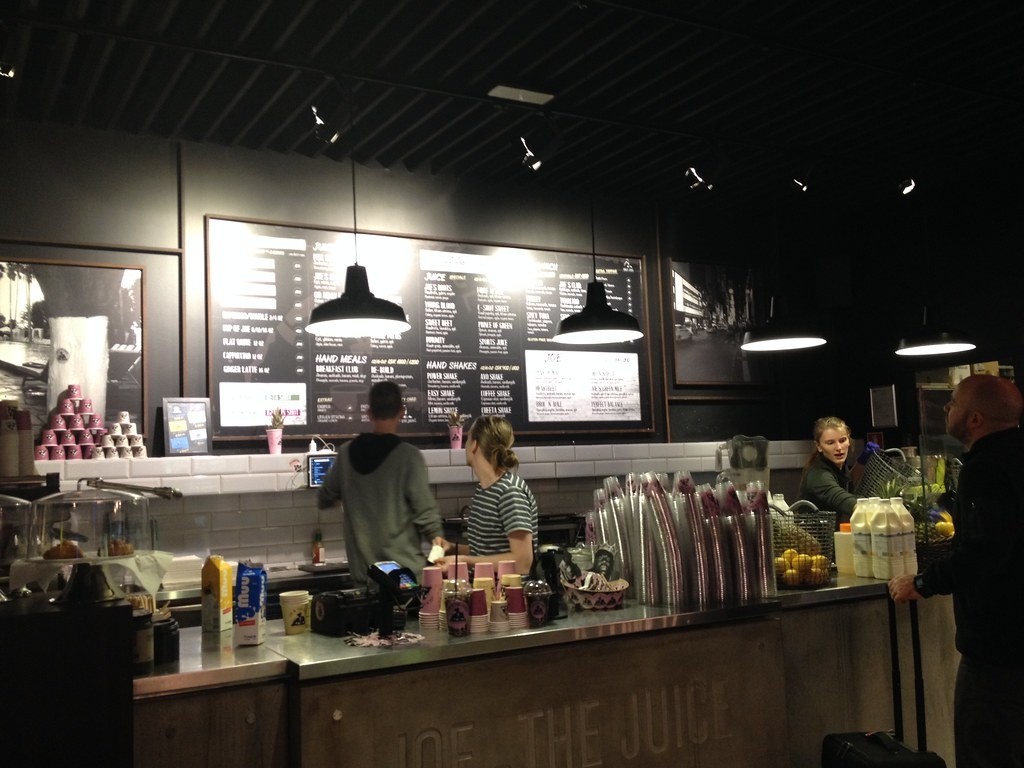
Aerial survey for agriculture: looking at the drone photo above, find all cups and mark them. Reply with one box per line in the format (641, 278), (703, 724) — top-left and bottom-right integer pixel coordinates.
(0, 383), (146, 478)
(279, 589), (313, 635)
(586, 470), (775, 609)
(418, 560), (554, 636)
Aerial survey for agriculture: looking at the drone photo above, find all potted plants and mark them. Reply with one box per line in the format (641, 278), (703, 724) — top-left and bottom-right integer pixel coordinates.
(449, 411), (468, 450)
(268, 409), (285, 458)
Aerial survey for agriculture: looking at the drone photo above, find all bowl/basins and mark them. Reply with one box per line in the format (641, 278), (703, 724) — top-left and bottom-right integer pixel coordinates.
(563, 578), (630, 610)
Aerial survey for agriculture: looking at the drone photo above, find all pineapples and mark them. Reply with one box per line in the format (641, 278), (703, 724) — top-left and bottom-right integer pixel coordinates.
(908, 494), (937, 542)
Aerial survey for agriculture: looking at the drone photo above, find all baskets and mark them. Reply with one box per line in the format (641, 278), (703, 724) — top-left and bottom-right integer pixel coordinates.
(851, 447), (925, 503)
(768, 499), (837, 588)
(915, 521), (957, 573)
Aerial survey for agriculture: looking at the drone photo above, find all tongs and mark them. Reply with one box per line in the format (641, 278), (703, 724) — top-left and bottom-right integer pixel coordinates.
(87, 479), (183, 499)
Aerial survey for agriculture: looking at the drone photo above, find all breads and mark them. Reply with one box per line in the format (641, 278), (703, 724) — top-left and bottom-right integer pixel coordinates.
(97, 538), (134, 556)
(42, 540), (83, 559)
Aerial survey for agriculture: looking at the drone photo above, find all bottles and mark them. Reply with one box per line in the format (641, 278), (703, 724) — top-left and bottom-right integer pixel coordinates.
(834, 496), (917, 580)
(770, 492), (795, 526)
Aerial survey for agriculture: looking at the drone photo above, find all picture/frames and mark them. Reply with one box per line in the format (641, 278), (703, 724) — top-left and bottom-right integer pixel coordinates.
(162, 399), (209, 457)
(870, 384), (898, 429)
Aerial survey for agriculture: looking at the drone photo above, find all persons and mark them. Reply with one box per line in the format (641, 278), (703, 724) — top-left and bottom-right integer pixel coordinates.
(886, 374), (1024, 768)
(431, 414), (538, 582)
(319, 381), (444, 588)
(796, 417), (860, 529)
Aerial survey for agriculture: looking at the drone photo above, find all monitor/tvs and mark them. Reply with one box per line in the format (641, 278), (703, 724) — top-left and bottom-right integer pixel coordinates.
(306, 454), (338, 488)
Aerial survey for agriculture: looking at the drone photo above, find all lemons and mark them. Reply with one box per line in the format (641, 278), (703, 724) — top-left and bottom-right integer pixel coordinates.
(934, 511), (954, 537)
(774, 548), (831, 586)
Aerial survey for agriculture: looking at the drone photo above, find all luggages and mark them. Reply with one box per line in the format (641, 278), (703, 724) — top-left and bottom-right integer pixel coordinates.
(820, 581), (949, 768)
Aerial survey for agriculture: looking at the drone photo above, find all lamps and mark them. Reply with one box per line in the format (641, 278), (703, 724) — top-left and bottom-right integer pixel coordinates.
(787, 156), (824, 192)
(682, 152), (717, 194)
(519, 121), (554, 173)
(893, 271), (979, 357)
(553, 4), (644, 344)
(309, 84), (407, 332)
(738, 240), (828, 354)
(880, 151), (918, 197)
(307, 91), (361, 167)
